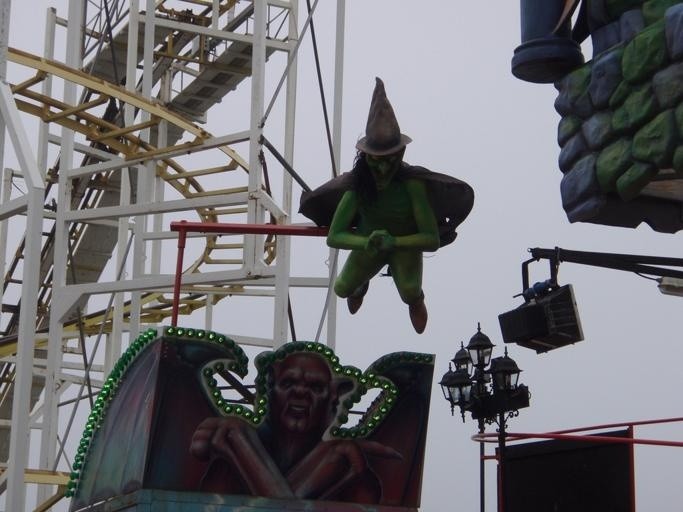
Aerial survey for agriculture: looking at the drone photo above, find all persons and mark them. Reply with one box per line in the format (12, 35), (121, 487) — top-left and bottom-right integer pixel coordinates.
(188, 352), (404, 505)
(326, 78), (443, 334)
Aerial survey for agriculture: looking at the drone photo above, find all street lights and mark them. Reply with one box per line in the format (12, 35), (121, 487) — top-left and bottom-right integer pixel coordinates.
(438, 322), (532, 512)
